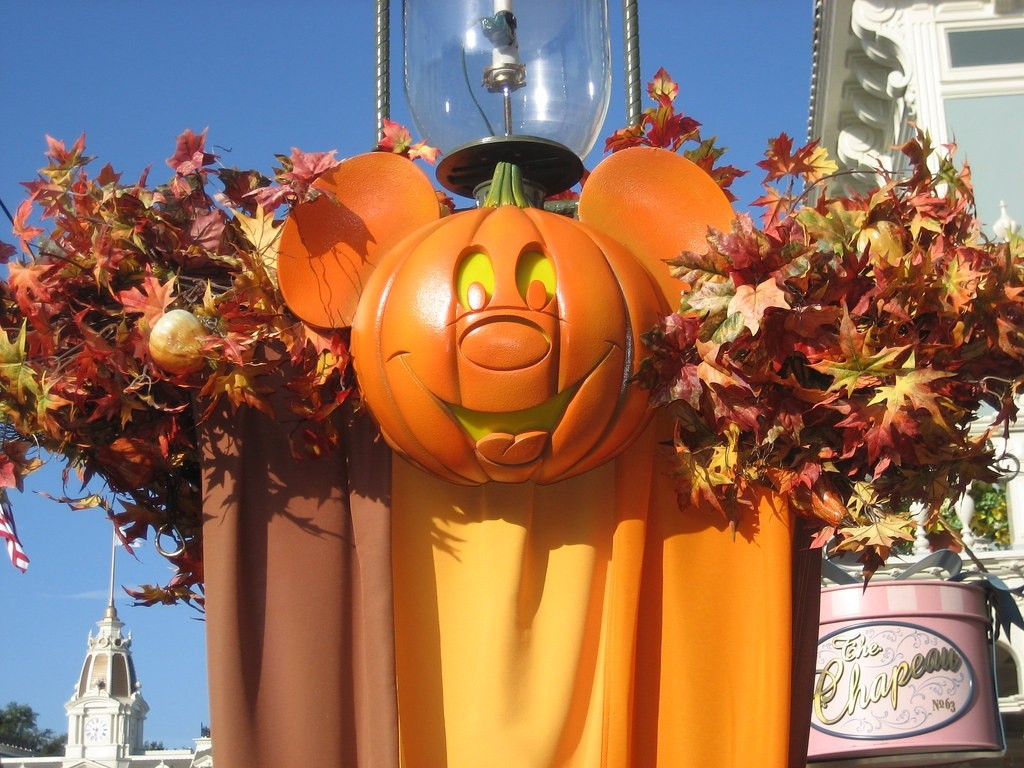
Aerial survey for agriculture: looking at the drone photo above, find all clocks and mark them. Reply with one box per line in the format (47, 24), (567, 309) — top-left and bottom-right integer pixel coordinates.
(85, 718), (108, 742)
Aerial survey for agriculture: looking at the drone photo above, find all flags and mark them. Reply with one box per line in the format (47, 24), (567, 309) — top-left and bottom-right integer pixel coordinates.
(0, 490), (31, 576)
(114, 523), (148, 547)
